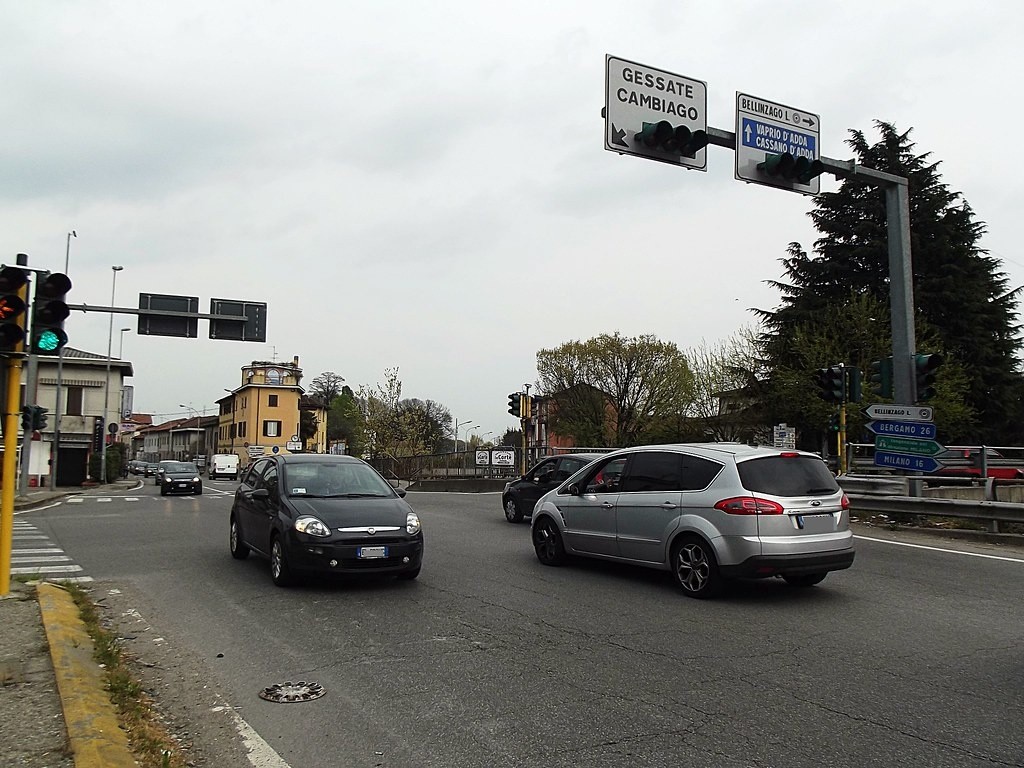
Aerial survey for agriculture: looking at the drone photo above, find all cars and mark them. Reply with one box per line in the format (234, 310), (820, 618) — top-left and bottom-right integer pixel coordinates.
(502, 453), (628, 523)
(128, 460), (204, 496)
(230, 453), (425, 586)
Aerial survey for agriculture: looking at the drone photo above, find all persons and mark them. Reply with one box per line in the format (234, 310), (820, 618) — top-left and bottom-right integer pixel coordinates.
(317, 465), (352, 494)
(539, 460), (570, 482)
(595, 461), (637, 494)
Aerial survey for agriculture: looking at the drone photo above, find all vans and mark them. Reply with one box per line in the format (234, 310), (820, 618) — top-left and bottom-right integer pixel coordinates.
(208, 454), (239, 481)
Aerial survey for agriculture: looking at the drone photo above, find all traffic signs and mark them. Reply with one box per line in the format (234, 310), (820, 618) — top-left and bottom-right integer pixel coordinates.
(858, 404), (948, 474)
(735, 91), (820, 196)
(605, 54), (707, 172)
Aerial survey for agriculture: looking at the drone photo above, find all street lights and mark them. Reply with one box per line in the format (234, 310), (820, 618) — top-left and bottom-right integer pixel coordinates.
(455, 418), (472, 459)
(465, 426), (480, 451)
(120, 328), (130, 359)
(100, 265), (123, 482)
(179, 405), (200, 466)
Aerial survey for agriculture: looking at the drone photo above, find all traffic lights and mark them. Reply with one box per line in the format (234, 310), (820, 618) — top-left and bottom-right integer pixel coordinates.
(765, 152), (824, 186)
(641, 121), (708, 159)
(0, 266), (71, 357)
(21, 406), (48, 430)
(913, 353), (942, 403)
(507, 394), (522, 417)
(818, 366), (845, 403)
(870, 355), (895, 400)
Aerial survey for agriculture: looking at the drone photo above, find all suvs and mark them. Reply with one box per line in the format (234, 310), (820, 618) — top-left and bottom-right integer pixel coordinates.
(927, 448), (1024, 487)
(529, 441), (856, 599)
